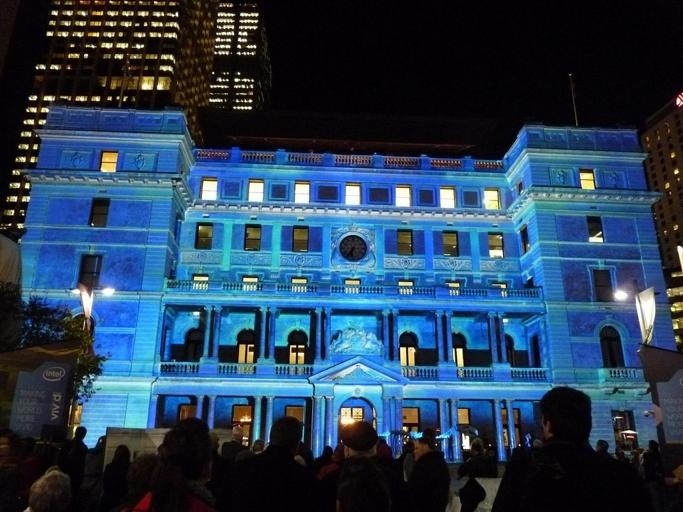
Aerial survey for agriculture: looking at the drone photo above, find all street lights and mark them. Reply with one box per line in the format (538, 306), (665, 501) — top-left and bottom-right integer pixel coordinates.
(612, 279), (666, 451)
(66, 272), (116, 438)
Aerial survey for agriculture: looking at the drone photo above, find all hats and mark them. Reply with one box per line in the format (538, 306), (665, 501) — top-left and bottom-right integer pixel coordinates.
(341, 421), (378, 451)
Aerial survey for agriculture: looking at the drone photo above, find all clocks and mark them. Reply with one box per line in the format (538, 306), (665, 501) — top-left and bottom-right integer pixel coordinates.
(339, 235), (367, 262)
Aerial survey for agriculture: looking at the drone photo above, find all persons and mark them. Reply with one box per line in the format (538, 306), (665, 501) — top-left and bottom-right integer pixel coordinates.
(0, 387), (683, 512)
